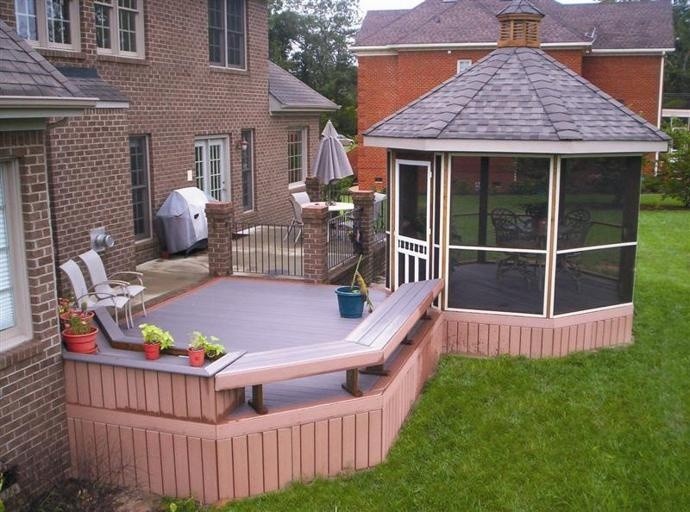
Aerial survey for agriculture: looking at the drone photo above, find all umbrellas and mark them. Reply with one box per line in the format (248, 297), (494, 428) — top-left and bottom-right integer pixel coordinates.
(311, 120), (355, 203)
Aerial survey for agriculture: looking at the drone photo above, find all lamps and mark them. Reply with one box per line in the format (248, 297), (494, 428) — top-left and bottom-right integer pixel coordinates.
(235, 136), (248, 151)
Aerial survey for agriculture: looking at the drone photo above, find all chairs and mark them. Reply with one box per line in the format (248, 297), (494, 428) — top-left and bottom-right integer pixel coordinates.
(291, 192), (310, 212)
(284, 201), (303, 243)
(59, 259), (130, 329)
(78, 249), (147, 327)
(491, 206), (591, 295)
(337, 192), (386, 242)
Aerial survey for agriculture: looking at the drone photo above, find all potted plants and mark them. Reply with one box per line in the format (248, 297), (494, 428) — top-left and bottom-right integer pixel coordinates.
(138, 323), (174, 360)
(60, 303), (95, 329)
(57, 298), (70, 313)
(62, 317), (101, 355)
(187, 331), (225, 367)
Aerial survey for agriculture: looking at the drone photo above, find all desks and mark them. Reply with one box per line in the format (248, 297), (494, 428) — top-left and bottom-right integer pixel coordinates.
(301, 202), (354, 229)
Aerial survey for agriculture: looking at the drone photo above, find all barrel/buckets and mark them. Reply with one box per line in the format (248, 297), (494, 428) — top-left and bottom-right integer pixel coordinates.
(335, 286), (365, 318)
